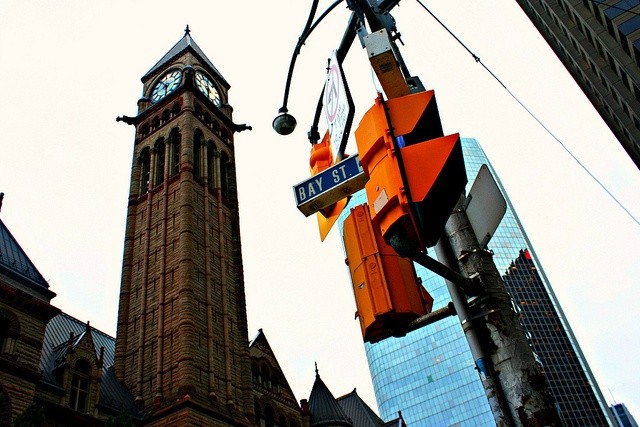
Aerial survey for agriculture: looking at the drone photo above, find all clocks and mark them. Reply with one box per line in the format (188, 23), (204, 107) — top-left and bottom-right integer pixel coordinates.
(194, 69), (223, 110)
(148, 67), (182, 104)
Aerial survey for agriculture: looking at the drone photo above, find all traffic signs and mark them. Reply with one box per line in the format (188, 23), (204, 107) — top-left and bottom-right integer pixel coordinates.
(293, 151), (366, 208)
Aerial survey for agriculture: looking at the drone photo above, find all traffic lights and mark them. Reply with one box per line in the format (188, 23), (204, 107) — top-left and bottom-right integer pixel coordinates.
(380, 88), (469, 253)
